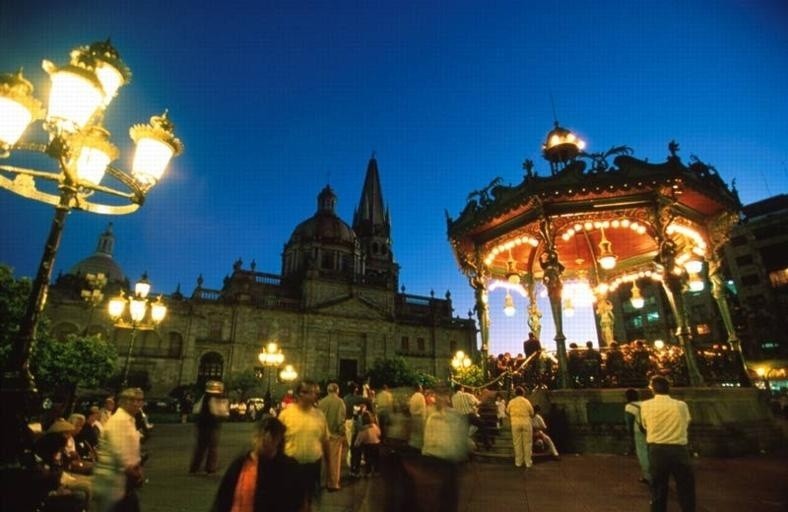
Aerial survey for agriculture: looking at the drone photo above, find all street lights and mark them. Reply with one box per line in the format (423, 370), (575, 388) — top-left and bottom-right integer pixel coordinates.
(258, 337), (285, 414)
(0, 36), (183, 411)
(105, 266), (171, 395)
(755, 364), (775, 399)
(451, 349), (472, 386)
(277, 363), (298, 393)
(74, 262), (110, 340)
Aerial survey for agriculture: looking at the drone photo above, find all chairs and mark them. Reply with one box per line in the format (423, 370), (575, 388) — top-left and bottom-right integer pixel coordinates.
(563, 348), (654, 388)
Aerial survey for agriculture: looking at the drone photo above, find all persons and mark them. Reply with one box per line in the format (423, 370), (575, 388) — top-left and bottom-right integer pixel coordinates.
(209, 415), (312, 510)
(177, 392), (194, 423)
(625, 389), (653, 484)
(494, 386), (559, 469)
(25, 384), (155, 509)
(486, 331), (559, 393)
(637, 370), (703, 509)
(187, 378), (232, 476)
(568, 340), (651, 389)
(352, 452), (480, 510)
(266, 377), (492, 492)
(678, 340), (741, 388)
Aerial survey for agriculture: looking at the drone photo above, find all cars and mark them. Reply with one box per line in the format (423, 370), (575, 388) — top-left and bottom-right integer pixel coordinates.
(71, 391), (264, 417)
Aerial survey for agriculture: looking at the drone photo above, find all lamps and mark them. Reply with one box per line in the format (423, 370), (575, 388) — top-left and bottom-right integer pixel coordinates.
(499, 220), (707, 317)
